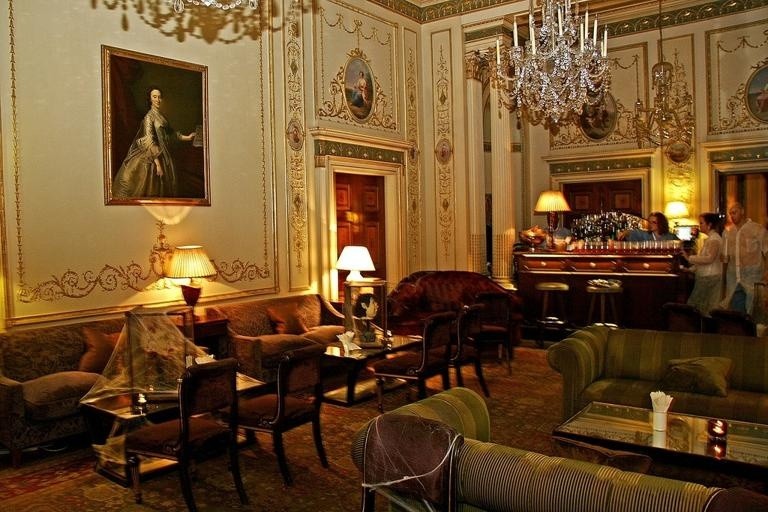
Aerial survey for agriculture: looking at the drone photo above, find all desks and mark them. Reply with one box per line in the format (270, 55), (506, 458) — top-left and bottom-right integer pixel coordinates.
(317, 332), (423, 408)
(78, 369), (268, 489)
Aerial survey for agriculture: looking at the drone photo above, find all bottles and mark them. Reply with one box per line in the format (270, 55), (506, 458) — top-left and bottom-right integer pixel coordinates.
(571, 210), (629, 241)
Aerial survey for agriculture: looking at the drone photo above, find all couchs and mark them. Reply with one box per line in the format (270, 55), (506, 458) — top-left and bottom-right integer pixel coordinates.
(0, 291), (192, 457)
(543, 320), (767, 417)
(348, 385), (767, 512)
(373, 268), (527, 361)
(208, 289), (383, 395)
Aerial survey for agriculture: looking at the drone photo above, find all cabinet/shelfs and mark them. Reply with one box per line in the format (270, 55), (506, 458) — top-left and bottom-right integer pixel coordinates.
(174, 312), (231, 359)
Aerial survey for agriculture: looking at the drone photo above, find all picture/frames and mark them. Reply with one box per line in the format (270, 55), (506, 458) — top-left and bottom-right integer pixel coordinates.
(743, 63), (767, 124)
(609, 190), (633, 211)
(340, 55), (378, 123)
(574, 192), (593, 212)
(96, 42), (213, 210)
(574, 86), (617, 142)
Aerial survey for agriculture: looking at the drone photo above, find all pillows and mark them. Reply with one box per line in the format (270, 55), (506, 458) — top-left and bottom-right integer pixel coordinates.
(656, 354), (739, 398)
(266, 299), (310, 336)
(76, 321), (122, 374)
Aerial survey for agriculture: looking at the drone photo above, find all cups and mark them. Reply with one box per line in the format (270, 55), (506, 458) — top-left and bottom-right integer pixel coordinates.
(579, 239), (682, 256)
(708, 418), (727, 437)
(706, 438), (726, 457)
(651, 410), (668, 433)
(651, 430), (667, 448)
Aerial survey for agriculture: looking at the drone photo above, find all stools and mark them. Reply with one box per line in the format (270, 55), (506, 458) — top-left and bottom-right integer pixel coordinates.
(532, 282), (571, 339)
(584, 282), (624, 325)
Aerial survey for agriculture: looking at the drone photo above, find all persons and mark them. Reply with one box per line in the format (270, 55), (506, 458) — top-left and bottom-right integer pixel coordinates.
(677, 212), (727, 317)
(720, 203), (767, 315)
(615, 213), (681, 242)
(355, 295), (381, 334)
(109, 86), (199, 198)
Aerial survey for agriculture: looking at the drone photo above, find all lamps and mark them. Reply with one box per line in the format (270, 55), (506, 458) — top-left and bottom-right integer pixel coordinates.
(625, 0), (698, 149)
(161, 243), (218, 311)
(470, 2), (612, 127)
(661, 200), (691, 231)
(530, 185), (572, 243)
(333, 244), (377, 290)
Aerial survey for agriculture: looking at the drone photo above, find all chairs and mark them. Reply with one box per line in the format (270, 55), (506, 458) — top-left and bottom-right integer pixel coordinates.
(651, 302), (702, 332)
(372, 310), (459, 413)
(704, 308), (756, 338)
(474, 292), (515, 378)
(125, 355), (249, 510)
(223, 339), (332, 489)
(454, 301), (490, 398)
(357, 412), (467, 512)
